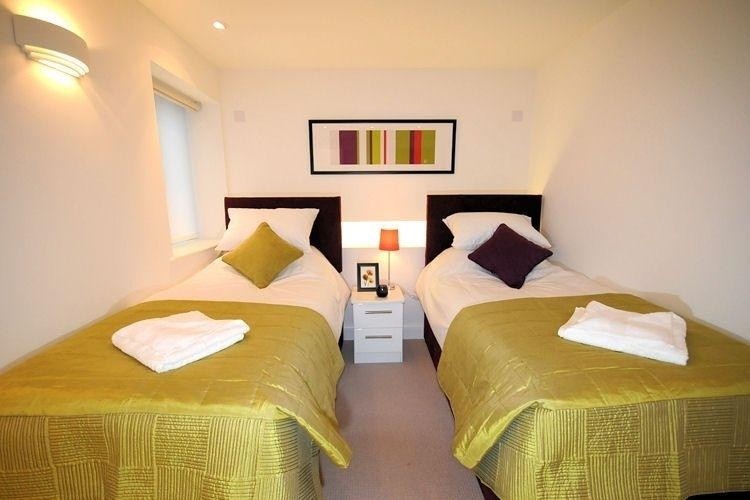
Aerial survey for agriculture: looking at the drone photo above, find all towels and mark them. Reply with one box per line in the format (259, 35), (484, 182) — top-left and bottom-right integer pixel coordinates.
(111, 310), (250, 374)
(557, 300), (689, 366)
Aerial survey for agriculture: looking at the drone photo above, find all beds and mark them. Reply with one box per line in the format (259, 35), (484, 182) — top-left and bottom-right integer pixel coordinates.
(0, 197), (353, 500)
(414, 195), (750, 500)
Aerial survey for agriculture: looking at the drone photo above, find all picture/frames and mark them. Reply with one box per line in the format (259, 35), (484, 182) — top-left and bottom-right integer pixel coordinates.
(309, 119), (457, 174)
(357, 263), (379, 292)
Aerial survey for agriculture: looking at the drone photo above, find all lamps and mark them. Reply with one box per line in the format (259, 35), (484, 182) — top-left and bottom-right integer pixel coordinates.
(379, 228), (400, 290)
(12, 13), (90, 78)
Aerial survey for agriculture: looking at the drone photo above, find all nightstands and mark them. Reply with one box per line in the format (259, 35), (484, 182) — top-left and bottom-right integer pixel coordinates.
(351, 285), (405, 364)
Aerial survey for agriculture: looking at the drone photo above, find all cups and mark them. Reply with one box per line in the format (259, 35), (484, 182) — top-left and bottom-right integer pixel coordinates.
(375, 284), (388, 297)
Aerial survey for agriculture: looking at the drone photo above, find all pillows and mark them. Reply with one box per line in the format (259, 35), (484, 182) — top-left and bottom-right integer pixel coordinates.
(215, 207), (320, 255)
(467, 223), (553, 290)
(222, 222), (304, 290)
(442, 211), (552, 251)
